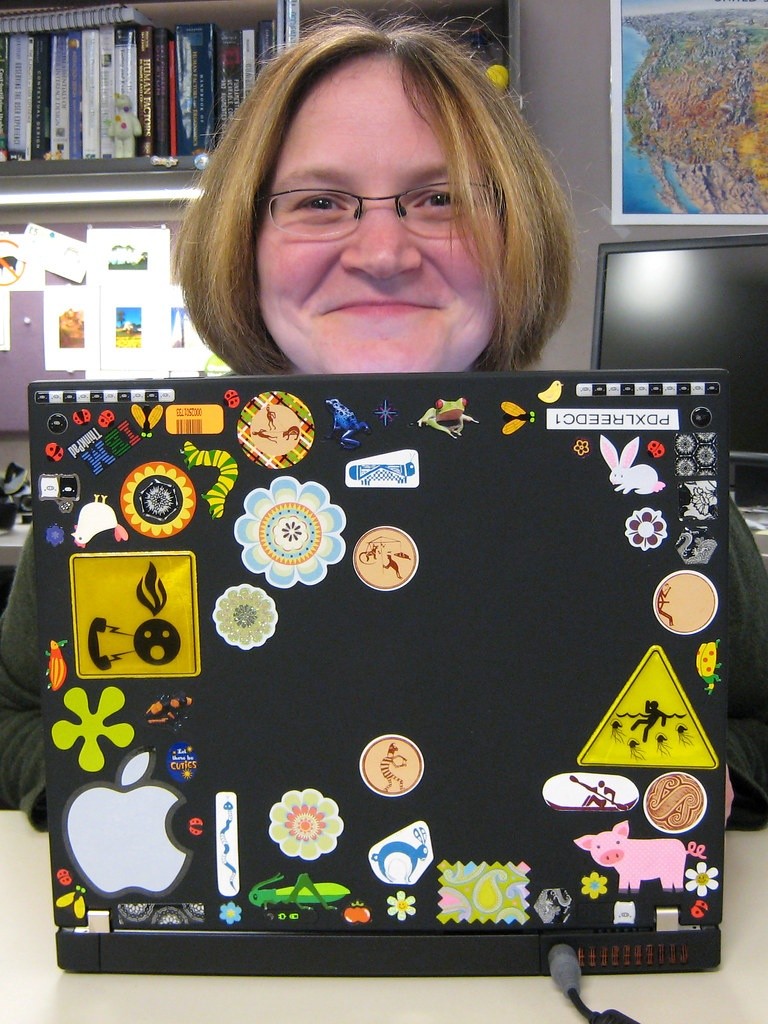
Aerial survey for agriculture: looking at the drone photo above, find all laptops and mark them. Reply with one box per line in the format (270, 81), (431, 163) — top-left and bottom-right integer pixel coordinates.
(27, 366), (728, 978)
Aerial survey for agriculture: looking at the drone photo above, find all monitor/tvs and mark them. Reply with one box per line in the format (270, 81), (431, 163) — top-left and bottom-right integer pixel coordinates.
(592, 233), (768, 509)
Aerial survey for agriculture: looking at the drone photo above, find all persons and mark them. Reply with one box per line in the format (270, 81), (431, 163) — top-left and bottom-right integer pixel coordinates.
(0, 9), (768, 833)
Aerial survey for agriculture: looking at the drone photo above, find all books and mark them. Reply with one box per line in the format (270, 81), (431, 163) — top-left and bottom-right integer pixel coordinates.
(0, 2), (278, 162)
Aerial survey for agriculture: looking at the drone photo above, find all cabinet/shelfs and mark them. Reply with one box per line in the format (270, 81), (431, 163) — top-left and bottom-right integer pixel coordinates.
(0, 0), (304, 211)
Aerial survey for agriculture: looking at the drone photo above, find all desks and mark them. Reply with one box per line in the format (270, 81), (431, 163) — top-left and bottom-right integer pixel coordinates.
(610, 1), (767, 225)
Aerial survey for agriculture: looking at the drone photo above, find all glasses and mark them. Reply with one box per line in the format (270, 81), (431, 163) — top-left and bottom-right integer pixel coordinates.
(256, 179), (505, 238)
